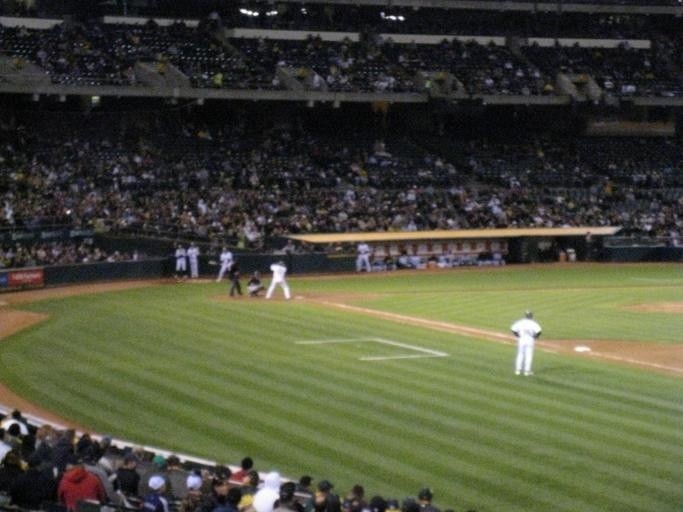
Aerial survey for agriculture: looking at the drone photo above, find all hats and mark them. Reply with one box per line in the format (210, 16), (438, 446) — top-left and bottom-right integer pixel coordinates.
(62, 455), (83, 464)
(187, 476), (202, 490)
(152, 456), (167, 468)
(149, 476), (164, 490)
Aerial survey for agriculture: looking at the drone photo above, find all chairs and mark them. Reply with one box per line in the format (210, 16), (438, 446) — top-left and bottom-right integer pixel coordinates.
(0, 20), (682, 252)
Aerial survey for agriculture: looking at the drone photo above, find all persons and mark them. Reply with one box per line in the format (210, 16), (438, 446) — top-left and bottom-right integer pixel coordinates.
(509, 308), (543, 378)
